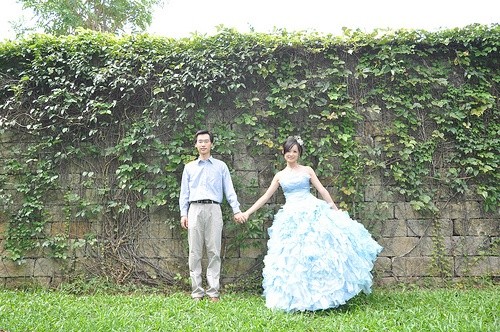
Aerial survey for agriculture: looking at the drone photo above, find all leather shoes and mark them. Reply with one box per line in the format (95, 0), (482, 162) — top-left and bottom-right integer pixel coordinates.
(194, 298), (202, 301)
(211, 297), (219, 302)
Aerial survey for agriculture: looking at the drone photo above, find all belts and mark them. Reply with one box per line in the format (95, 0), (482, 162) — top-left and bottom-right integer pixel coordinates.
(190, 199), (219, 204)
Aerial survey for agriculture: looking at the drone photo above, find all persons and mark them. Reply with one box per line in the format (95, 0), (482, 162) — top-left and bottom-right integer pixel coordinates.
(237, 135), (383, 313)
(179, 130), (246, 302)
